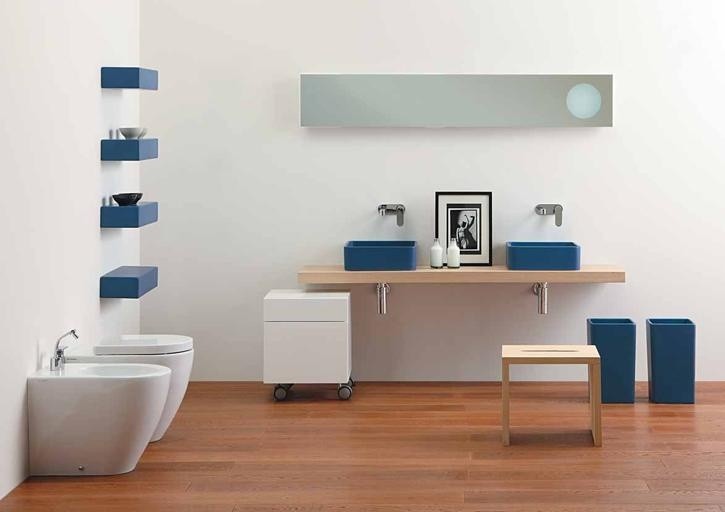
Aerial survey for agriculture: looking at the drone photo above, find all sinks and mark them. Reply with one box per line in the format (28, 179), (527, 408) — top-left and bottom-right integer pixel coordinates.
(343, 241), (417, 271)
(27, 363), (171, 476)
(506, 241), (580, 271)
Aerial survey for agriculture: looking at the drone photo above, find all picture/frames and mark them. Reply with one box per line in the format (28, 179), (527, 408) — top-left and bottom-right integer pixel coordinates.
(435, 191), (493, 267)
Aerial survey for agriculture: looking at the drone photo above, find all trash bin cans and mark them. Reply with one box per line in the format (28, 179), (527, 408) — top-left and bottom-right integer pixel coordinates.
(646, 318), (696, 405)
(586, 318), (636, 404)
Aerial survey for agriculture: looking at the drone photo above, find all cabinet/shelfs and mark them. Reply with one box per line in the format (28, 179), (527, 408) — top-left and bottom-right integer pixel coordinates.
(263, 288), (353, 401)
(101, 66), (159, 298)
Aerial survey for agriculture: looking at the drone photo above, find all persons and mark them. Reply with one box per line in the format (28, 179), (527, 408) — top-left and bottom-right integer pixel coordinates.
(457, 214), (469, 249)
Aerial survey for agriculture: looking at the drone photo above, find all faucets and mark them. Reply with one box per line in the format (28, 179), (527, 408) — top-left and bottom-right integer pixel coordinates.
(50, 329), (78, 371)
(379, 205), (388, 217)
(539, 207), (547, 216)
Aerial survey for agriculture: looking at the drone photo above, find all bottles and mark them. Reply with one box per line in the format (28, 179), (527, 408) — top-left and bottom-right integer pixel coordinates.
(447, 238), (460, 269)
(430, 238), (443, 268)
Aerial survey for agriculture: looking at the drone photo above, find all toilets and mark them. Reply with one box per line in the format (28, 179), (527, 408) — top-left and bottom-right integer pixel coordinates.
(78, 334), (195, 443)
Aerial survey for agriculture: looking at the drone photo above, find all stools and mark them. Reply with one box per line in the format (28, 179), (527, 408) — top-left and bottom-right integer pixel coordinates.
(502, 344), (602, 447)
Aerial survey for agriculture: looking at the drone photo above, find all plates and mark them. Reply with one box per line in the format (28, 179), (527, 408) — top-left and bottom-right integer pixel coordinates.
(566, 84), (601, 119)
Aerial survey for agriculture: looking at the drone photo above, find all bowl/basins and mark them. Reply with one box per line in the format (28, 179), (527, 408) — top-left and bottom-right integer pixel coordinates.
(112, 193), (143, 206)
(120, 127), (148, 139)
